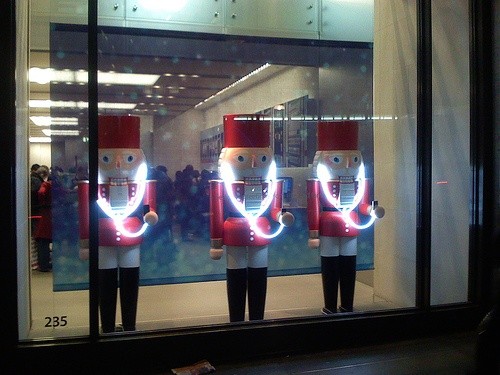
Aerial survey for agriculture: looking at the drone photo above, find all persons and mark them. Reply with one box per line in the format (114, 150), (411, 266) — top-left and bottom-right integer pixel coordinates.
(30, 161), (215, 273)
